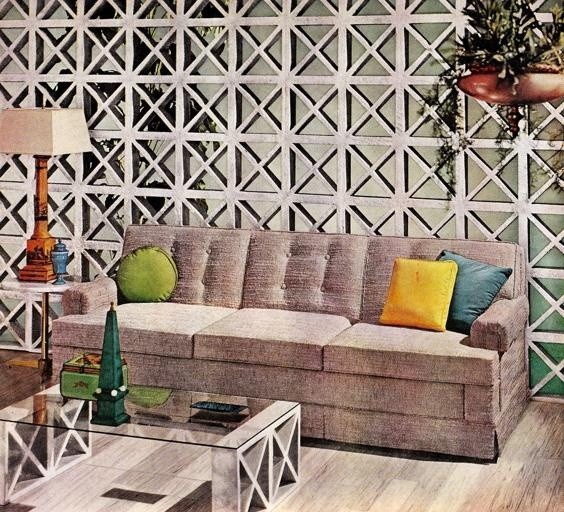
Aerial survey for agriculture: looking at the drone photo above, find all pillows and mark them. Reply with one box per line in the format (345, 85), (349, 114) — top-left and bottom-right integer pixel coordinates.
(432, 245), (514, 337)
(379, 251), (459, 336)
(110, 243), (177, 306)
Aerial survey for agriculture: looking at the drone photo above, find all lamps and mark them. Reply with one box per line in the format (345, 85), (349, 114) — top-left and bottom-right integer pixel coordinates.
(0, 105), (96, 283)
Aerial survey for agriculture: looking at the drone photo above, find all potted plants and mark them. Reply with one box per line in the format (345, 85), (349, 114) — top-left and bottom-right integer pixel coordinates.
(413, 1), (563, 213)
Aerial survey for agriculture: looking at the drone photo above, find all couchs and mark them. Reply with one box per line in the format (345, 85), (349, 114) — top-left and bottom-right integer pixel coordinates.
(50, 218), (533, 469)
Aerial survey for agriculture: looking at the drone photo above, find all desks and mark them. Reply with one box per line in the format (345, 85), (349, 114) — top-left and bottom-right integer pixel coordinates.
(0, 273), (81, 392)
